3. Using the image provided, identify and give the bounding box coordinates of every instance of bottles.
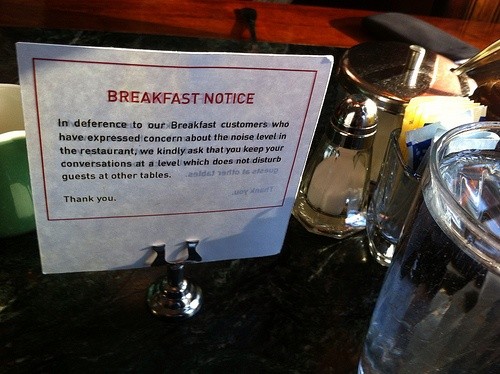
[301,93,378,218]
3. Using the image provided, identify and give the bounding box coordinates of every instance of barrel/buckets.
[357,119,500,374]
[331,39,467,198]
[366,127,438,267]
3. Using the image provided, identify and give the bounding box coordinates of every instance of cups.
[0,83,33,234]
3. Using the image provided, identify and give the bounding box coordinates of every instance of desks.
[0,0,500,51]
